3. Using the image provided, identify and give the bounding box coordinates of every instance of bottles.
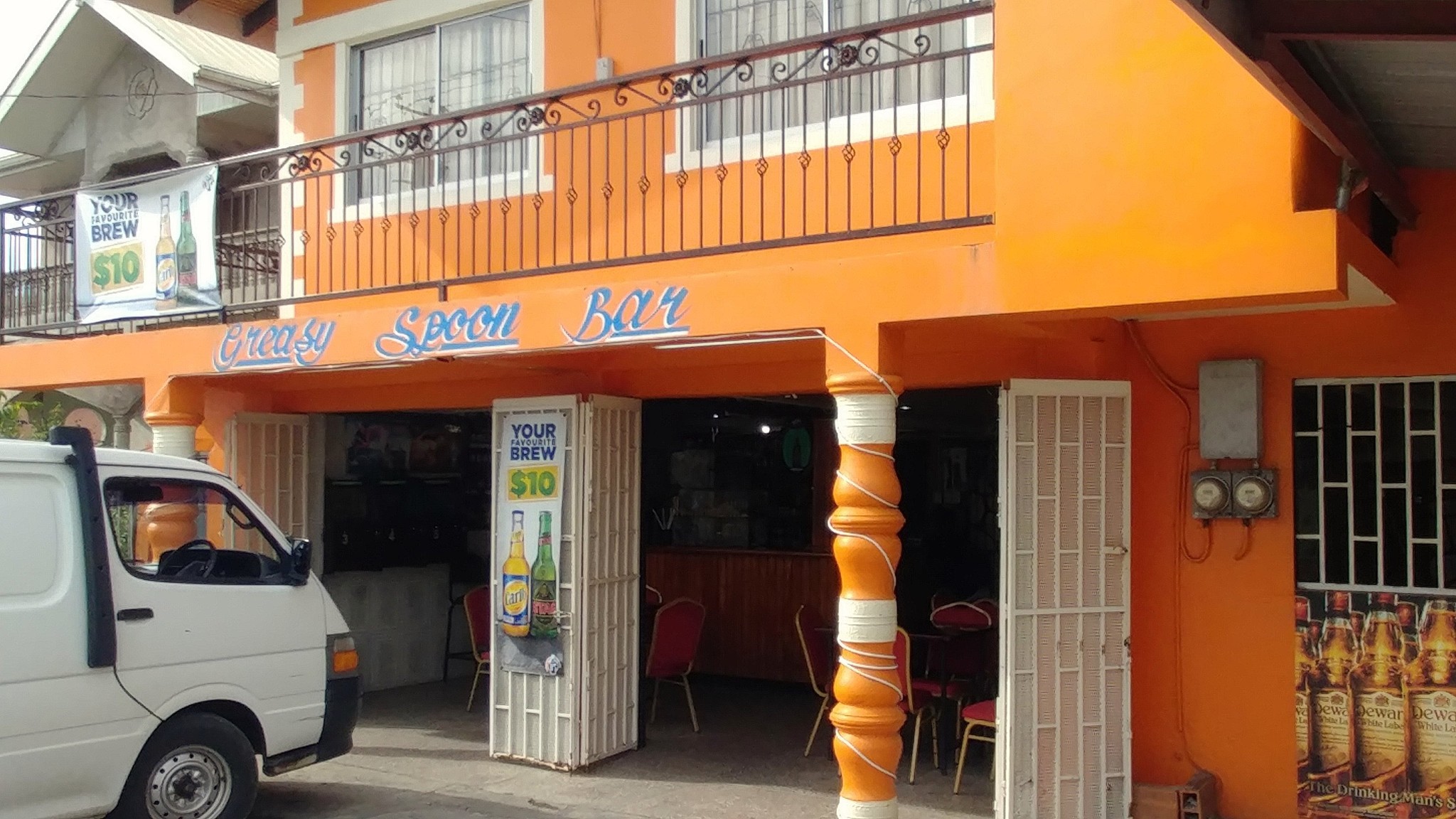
[155,195,177,310]
[1397,600,1420,663]
[530,510,557,638]
[1311,592,1352,810]
[1350,612,1365,664]
[502,510,529,637]
[1294,595,1311,794]
[1401,601,1456,819]
[1311,620,1323,667]
[1347,591,1408,819]
[176,191,198,305]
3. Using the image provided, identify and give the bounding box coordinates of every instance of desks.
[815,625,996,775]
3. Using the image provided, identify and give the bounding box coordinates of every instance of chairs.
[796,603,830,757]
[463,584,490,711]
[645,597,706,731]
[892,626,939,785]
[929,603,986,765]
[645,585,663,653]
[953,695,999,795]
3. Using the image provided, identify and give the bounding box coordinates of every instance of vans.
[0,426,362,819]
